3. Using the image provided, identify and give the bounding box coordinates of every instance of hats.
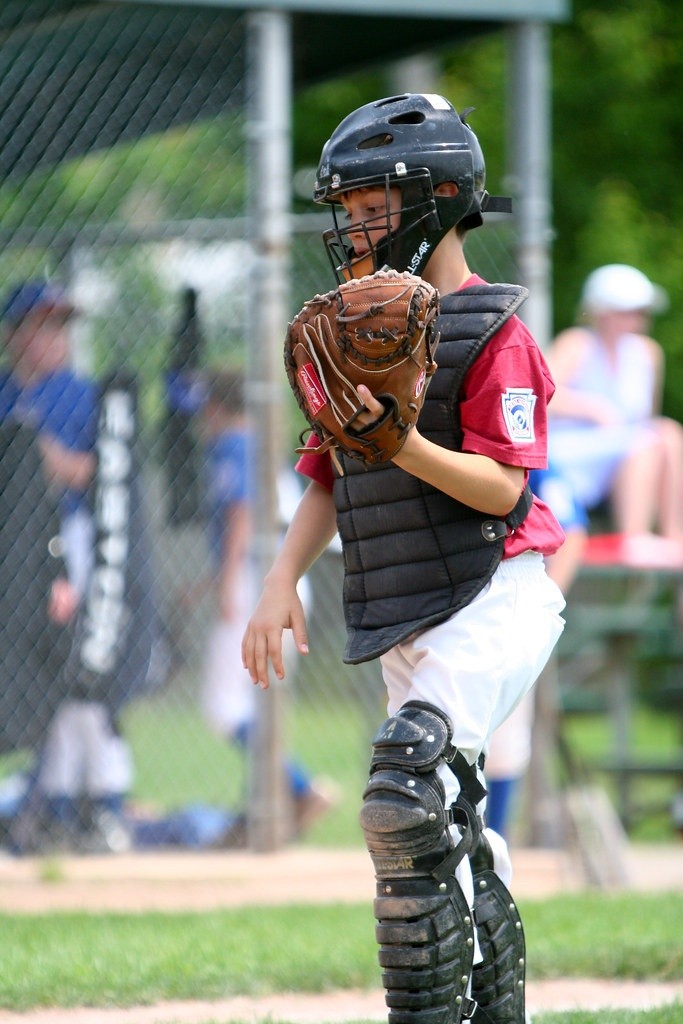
[584,264,655,311]
[0,280,82,324]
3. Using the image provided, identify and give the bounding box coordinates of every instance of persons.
[177,369,336,829]
[484,263,683,845]
[0,278,139,850]
[241,93,565,1024]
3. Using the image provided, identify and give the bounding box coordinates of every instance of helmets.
[314,92,513,287]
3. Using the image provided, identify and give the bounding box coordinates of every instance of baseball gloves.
[278,273,434,463]
